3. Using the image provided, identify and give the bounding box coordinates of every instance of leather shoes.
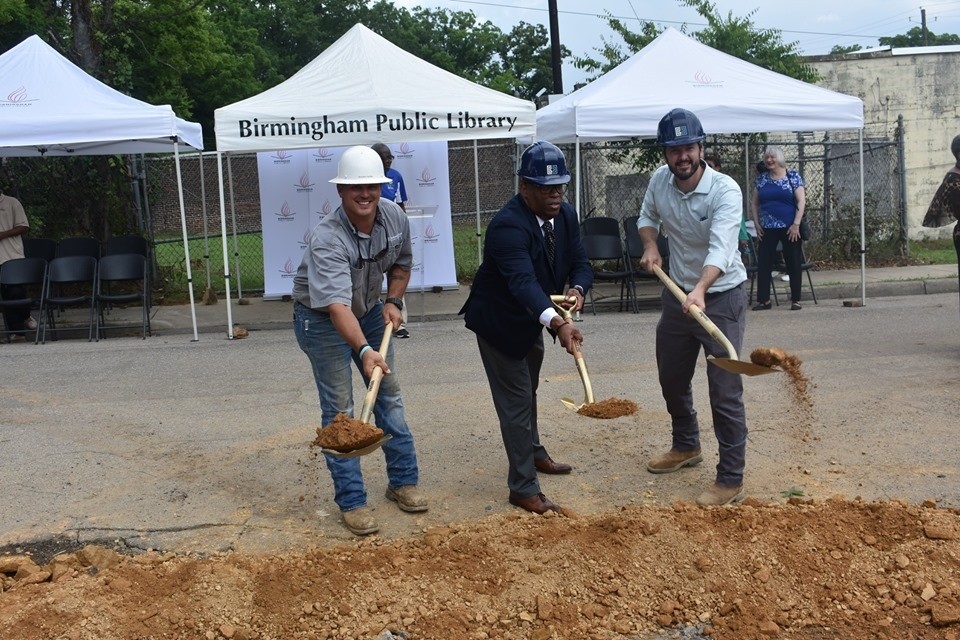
[508,491,559,515]
[536,456,571,474]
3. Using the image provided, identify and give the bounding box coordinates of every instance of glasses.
[536,183,568,194]
[383,155,395,160]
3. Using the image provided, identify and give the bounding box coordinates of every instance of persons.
[751,147,806,311]
[457,142,596,512]
[292,146,429,534]
[635,108,748,506]
[0,186,37,340]
[922,135,960,303]
[371,143,410,338]
[705,156,750,259]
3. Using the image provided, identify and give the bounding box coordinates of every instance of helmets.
[657,108,706,147]
[328,144,393,185]
[516,141,571,185]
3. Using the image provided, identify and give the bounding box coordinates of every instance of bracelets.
[359,344,372,359]
[545,322,569,344]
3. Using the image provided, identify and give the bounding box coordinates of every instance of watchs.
[385,298,403,310]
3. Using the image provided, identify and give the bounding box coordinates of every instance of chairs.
[744,220,819,308]
[568,216,637,316]
[56,235,105,315]
[41,254,102,346]
[622,216,670,314]
[94,252,152,342]
[0,256,56,345]
[23,237,59,316]
[102,234,152,316]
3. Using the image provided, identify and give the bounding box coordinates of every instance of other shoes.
[343,506,379,535]
[25,316,37,329]
[752,302,771,310]
[647,447,703,473]
[696,486,745,507]
[393,329,410,338]
[791,302,802,310]
[386,484,429,512]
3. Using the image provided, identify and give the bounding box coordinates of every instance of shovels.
[549,295,638,420]
[652,261,779,377]
[320,318,392,455]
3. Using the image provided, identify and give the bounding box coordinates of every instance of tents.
[214,22,538,341]
[515,28,866,323]
[0,34,217,342]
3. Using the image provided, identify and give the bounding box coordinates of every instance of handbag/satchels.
[800,215,811,241]
